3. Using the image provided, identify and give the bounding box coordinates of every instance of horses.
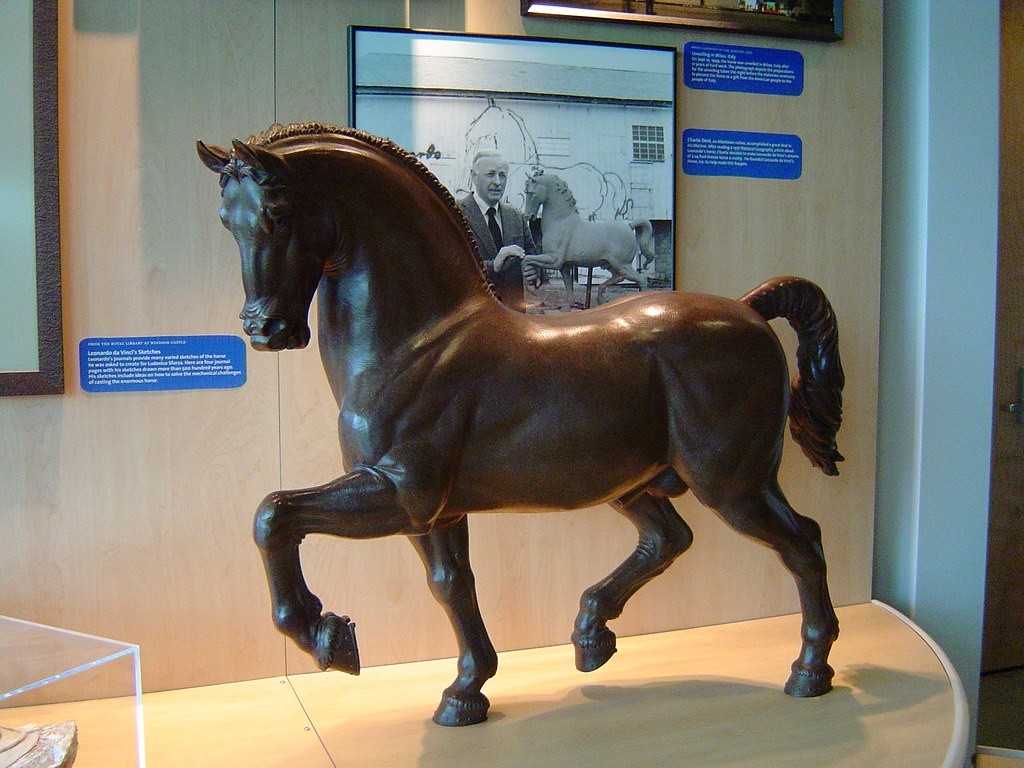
[193,121,848,728]
[523,170,654,310]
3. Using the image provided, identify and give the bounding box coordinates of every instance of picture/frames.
[0,0,72,398]
[519,0,844,46]
[346,24,678,318]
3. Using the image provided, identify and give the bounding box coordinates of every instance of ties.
[485,208,503,253]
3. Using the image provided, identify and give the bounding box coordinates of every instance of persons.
[458,151,544,314]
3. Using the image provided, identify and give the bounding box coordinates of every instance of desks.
[0,598,976,768]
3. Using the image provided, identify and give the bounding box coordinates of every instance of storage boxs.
[0,614,148,768]
